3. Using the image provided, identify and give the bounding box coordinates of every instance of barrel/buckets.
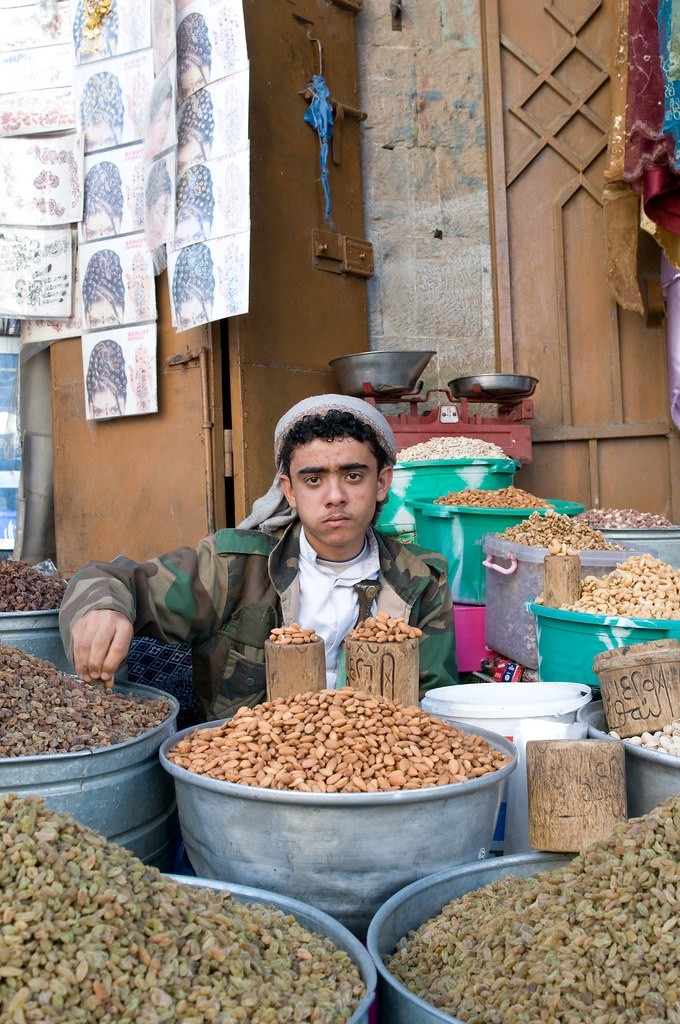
[0,459,680,1024]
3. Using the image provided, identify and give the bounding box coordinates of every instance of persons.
[59,393,460,724]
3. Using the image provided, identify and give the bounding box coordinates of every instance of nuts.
[0,433,680,1024]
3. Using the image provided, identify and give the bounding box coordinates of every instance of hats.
[273,394,397,481]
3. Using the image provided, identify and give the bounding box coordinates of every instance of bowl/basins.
[327,350,437,397]
[447,373,539,399]
